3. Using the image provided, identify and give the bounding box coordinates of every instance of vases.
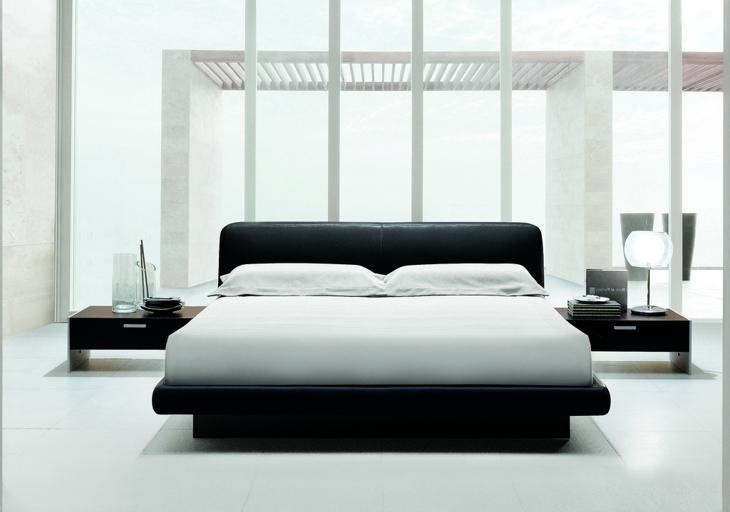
[110,252,156,315]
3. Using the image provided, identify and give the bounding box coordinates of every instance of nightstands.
[552,307,693,376]
[65,305,208,373]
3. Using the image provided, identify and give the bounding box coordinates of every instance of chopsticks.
[139,240,152,304]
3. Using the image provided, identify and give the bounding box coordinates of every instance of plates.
[574,296,611,304]
[140,304,183,315]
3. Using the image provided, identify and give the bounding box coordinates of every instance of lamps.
[623,230,673,317]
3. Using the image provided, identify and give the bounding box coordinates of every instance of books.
[566,298,623,318]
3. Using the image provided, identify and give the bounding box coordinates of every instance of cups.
[136,260,157,310]
[110,253,137,315]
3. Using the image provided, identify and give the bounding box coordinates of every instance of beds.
[151,223,610,452]
